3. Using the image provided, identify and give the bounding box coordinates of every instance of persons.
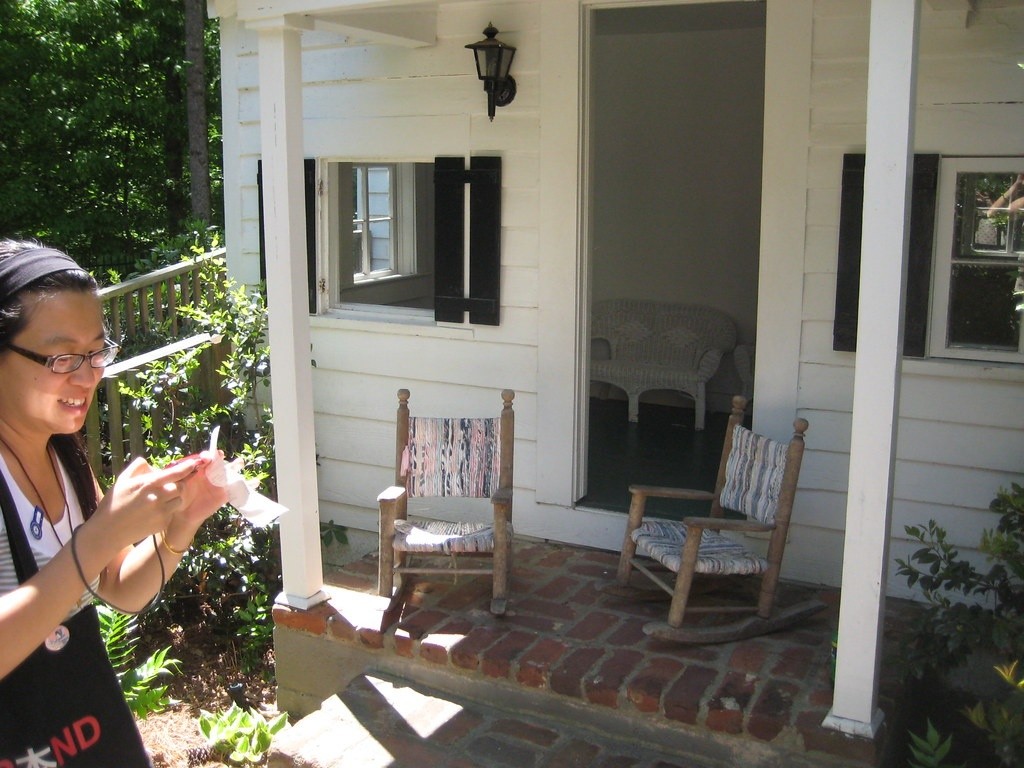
[0,243,234,768]
[986,174,1024,294]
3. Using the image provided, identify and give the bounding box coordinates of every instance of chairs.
[735,344,754,411]
[376,389,516,616]
[603,396,829,643]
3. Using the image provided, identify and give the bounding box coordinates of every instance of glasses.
[0,336,122,374]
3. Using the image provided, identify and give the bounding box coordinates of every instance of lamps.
[464,21,517,121]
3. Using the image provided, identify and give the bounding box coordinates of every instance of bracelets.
[1000,191,1010,203]
[159,529,195,556]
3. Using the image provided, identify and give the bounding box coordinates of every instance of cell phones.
[166,457,212,479]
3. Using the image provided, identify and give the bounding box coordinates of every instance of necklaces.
[0,438,73,548]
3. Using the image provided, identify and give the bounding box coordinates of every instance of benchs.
[590,298,739,433]
